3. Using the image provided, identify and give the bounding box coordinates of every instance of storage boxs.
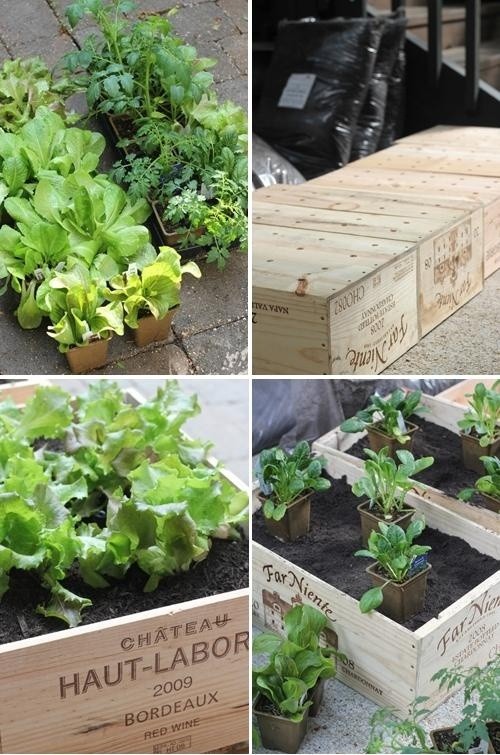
[432,379,500,417]
[252,448,500,723]
[348,143,500,278]
[396,122,500,141]
[253,184,420,375]
[304,166,484,341]
[311,389,500,539]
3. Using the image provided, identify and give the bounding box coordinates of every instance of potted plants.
[352,446,435,551]
[253,602,348,717]
[338,385,431,466]
[0,386,249,753]
[456,379,499,477]
[254,441,332,541]
[355,517,433,624]
[252,653,313,753]
[459,453,499,512]
